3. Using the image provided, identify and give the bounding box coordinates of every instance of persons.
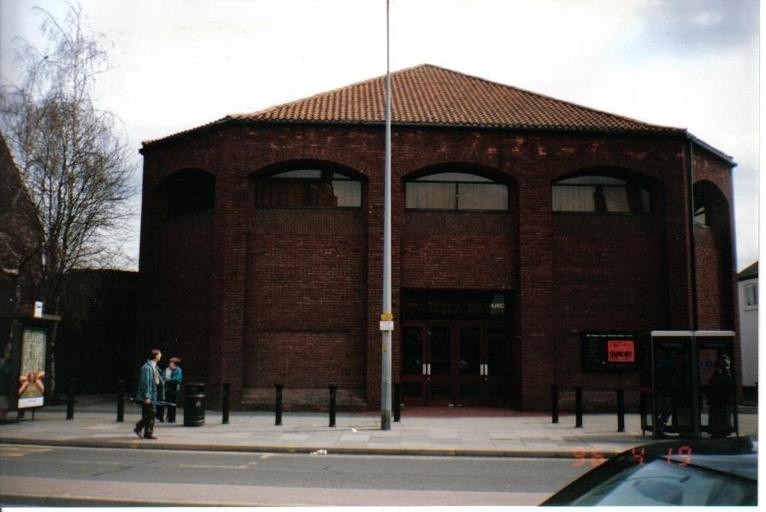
[160,357,183,422]
[133,349,162,439]
[18,371,46,396]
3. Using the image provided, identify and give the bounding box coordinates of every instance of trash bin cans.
[183,383,207,426]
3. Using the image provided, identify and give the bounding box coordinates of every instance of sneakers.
[133,428,158,439]
